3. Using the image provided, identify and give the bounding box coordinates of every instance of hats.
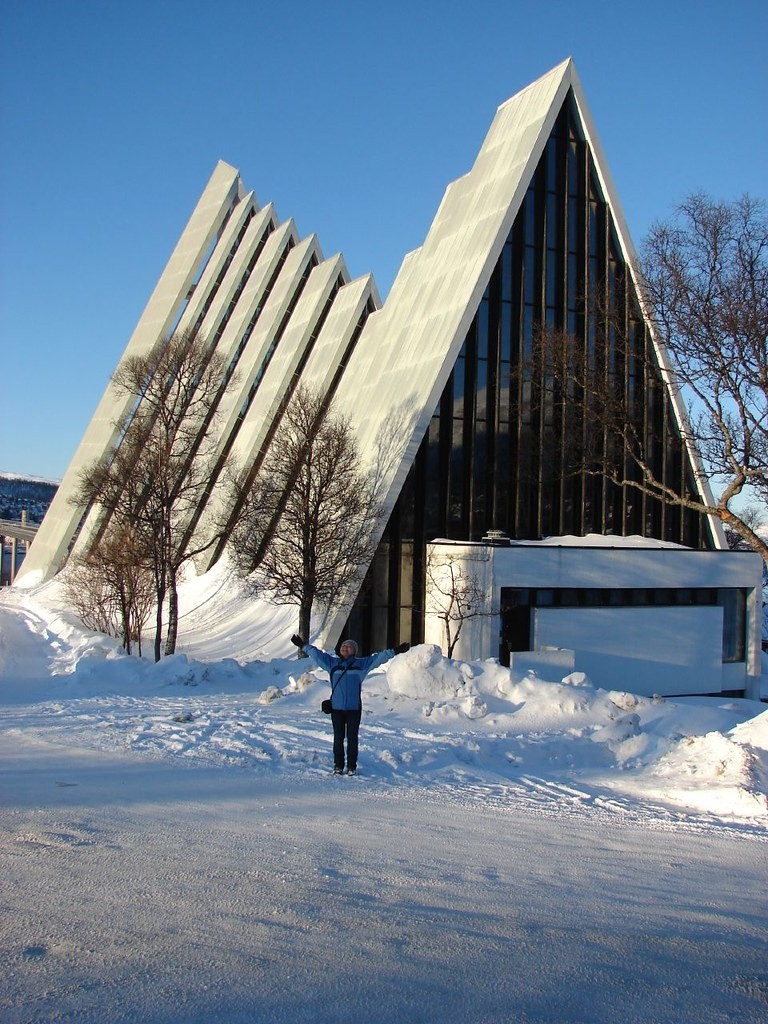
[342,639,358,657]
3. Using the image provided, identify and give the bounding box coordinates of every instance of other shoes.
[347,768,356,775]
[332,764,343,778]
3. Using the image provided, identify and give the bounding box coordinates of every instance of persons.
[291,634,410,775]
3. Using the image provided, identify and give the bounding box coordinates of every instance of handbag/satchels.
[321,699,332,714]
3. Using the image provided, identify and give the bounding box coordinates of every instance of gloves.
[290,634,303,648]
[393,641,410,655]
